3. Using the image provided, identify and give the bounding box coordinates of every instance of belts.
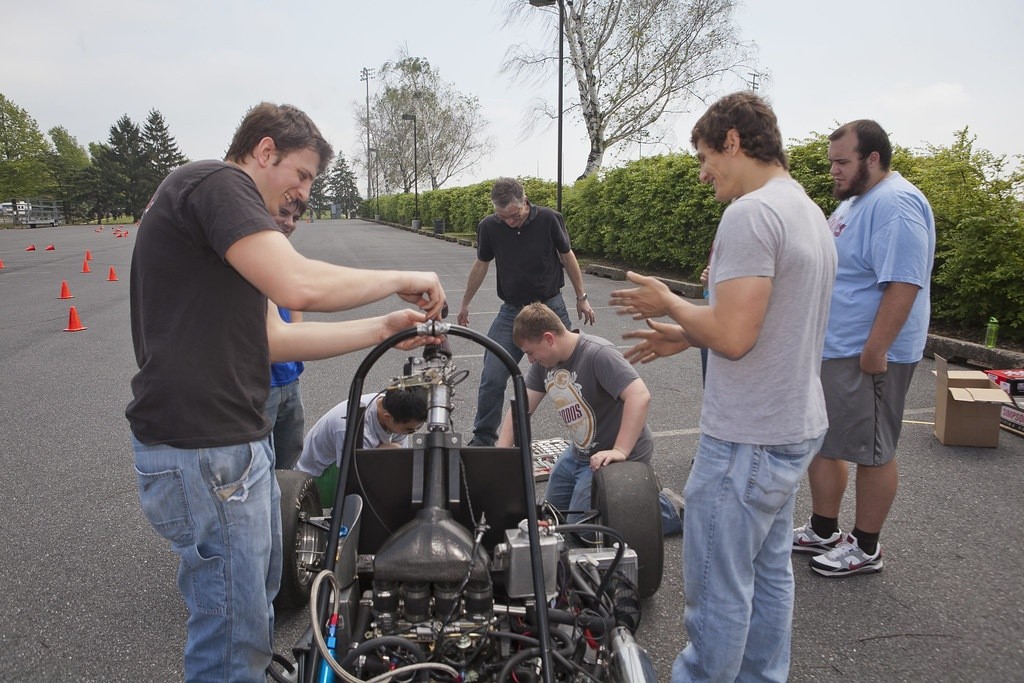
[505,291,561,309]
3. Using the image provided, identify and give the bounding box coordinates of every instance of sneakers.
[792,515,843,555]
[808,532,883,577]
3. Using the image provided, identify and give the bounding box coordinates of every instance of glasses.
[494,208,521,219]
[397,422,424,435]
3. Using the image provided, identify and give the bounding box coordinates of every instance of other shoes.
[467,435,494,446]
[662,487,685,531]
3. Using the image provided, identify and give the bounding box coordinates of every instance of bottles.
[985,316,999,347]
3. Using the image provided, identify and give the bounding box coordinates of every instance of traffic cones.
[120,224,123,227]
[85,249,94,260]
[62,306,88,332]
[124,230,128,234]
[80,259,92,273]
[57,280,74,299]
[113,229,121,234]
[124,232,126,236]
[46,244,55,250]
[107,266,119,281]
[0,259,4,269]
[117,233,122,237]
[95,224,103,232]
[25,245,35,251]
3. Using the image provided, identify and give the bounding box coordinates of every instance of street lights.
[370,148,379,215]
[361,67,376,199]
[529,0,564,214]
[402,114,418,220]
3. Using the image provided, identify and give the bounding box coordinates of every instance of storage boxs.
[983,368,1024,395]
[930,352,1013,447]
[1000,396,1024,435]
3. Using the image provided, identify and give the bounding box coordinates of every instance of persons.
[266,198,308,469]
[496,299,686,545]
[790,119,936,579]
[700,266,710,390]
[457,178,595,446]
[293,385,429,510]
[609,92,836,683]
[125,101,334,683]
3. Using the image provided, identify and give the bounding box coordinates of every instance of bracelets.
[576,293,587,301]
